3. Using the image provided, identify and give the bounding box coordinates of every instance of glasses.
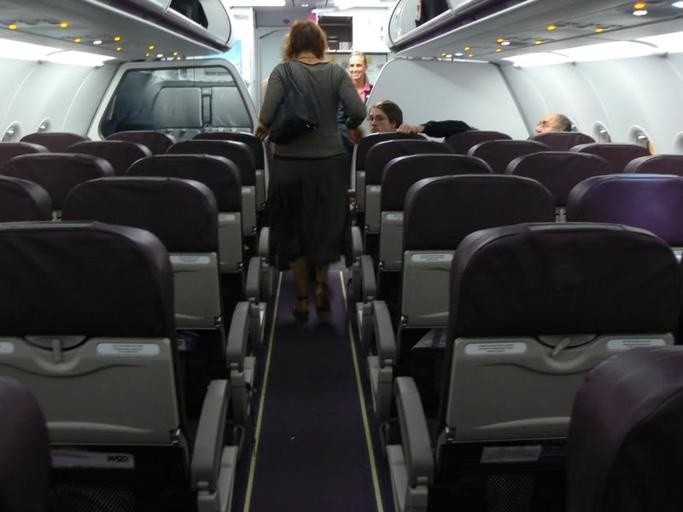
[367,117,387,121]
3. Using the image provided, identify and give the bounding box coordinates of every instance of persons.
[534,113,571,133]
[396,119,479,138]
[307,100,354,280]
[254,19,366,316]
[346,50,372,106]
[366,99,403,132]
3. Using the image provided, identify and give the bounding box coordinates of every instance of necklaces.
[295,54,320,63]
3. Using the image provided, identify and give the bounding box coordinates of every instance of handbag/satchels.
[268,85,319,145]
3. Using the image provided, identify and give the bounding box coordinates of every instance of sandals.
[293,295,310,322]
[315,281,331,309]
